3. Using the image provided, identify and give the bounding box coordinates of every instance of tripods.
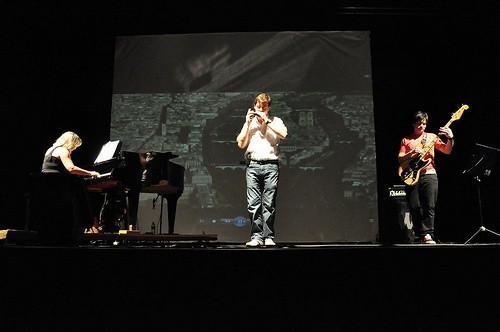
[465,150,500,245]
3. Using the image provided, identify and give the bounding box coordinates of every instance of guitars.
[397,103,469,186]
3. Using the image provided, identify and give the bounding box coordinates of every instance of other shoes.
[420,234,436,243]
[245,233,264,246]
[265,238,276,245]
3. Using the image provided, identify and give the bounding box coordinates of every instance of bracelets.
[448,137,454,140]
[265,120,271,125]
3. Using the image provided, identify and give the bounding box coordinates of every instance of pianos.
[76,140,184,235]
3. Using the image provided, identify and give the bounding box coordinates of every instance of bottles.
[151,222,155,234]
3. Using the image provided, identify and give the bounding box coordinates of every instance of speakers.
[384,196,417,244]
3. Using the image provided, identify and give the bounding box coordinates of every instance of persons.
[43,131,109,233]
[237,93,287,245]
[399,111,454,243]
